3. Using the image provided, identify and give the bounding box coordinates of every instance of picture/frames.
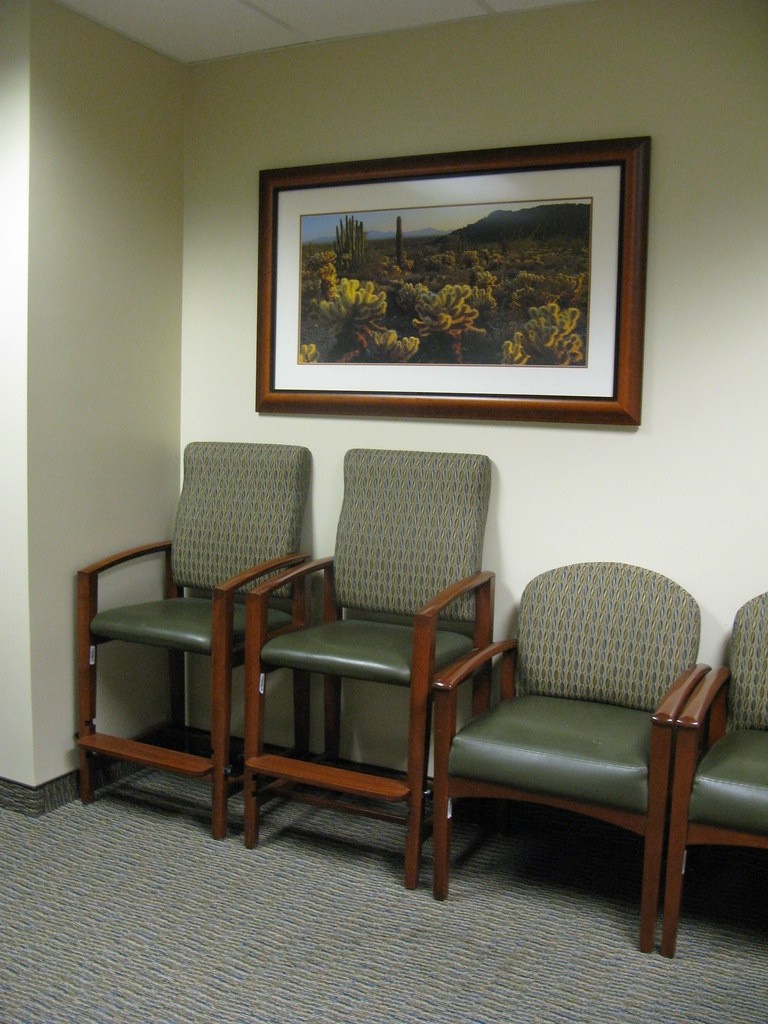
[253,137,650,427]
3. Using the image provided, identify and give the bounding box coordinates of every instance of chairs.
[429,561,713,953]
[243,449,494,894]
[73,440,313,840]
[663,586,768,958]
